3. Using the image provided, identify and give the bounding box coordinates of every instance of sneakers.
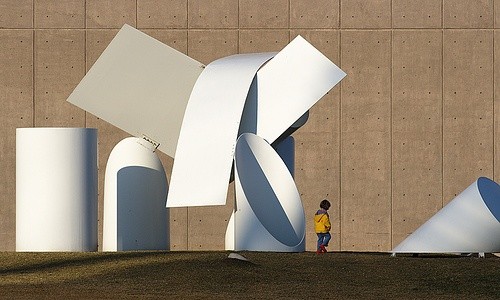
[320,245,327,252]
[317,251,325,254]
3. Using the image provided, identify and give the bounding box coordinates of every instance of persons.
[313,199,332,254]
